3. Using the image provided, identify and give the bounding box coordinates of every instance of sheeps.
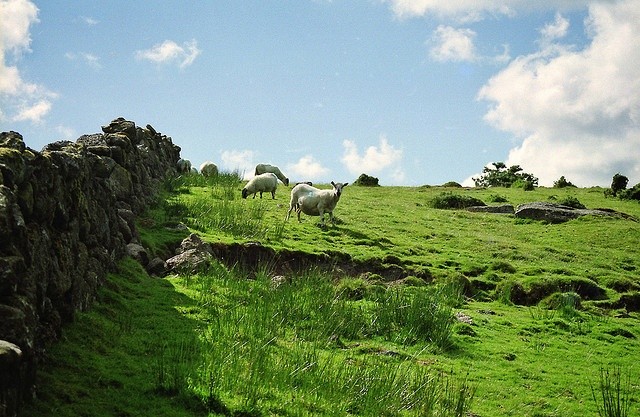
[242,173,278,199]
[255,164,289,186]
[176,159,191,175]
[286,181,348,229]
[200,161,218,177]
